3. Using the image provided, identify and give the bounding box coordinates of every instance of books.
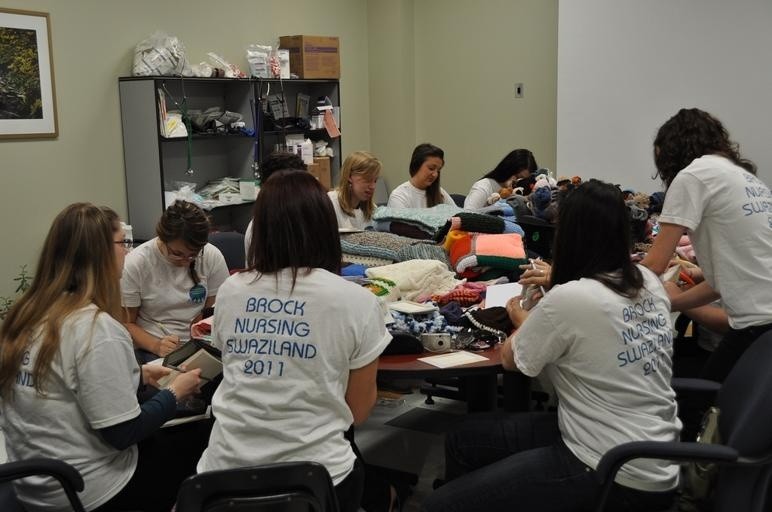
[154,346,222,404]
[163,175,260,210]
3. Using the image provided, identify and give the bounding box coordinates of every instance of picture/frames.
[0,8,59,141]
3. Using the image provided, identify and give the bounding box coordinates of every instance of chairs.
[171,462,339,512]
[0,457,86,512]
[581,331,772,512]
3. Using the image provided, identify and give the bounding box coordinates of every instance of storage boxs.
[281,35,340,79]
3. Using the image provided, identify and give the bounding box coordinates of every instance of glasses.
[163,240,206,266]
[113,237,134,250]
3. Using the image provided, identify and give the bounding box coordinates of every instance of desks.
[197,283,695,464]
[308,156,332,194]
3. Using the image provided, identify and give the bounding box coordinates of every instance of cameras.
[422,332,451,351]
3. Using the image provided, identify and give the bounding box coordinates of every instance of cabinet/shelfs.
[121,76,343,251]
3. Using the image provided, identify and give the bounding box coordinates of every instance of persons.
[415,179,680,507]
[635,108,772,422]
[243,152,314,268]
[462,149,540,213]
[326,151,387,234]
[384,143,459,214]
[119,202,229,356]
[665,264,736,378]
[192,169,393,508]
[0,204,204,507]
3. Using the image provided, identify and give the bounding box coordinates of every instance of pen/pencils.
[680,272,696,288]
[528,258,545,296]
[157,322,170,335]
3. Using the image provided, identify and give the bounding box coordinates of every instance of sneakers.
[378,373,411,395]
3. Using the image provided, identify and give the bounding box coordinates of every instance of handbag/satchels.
[681,405,723,503]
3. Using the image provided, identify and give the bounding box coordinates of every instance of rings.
[538,269,545,277]
